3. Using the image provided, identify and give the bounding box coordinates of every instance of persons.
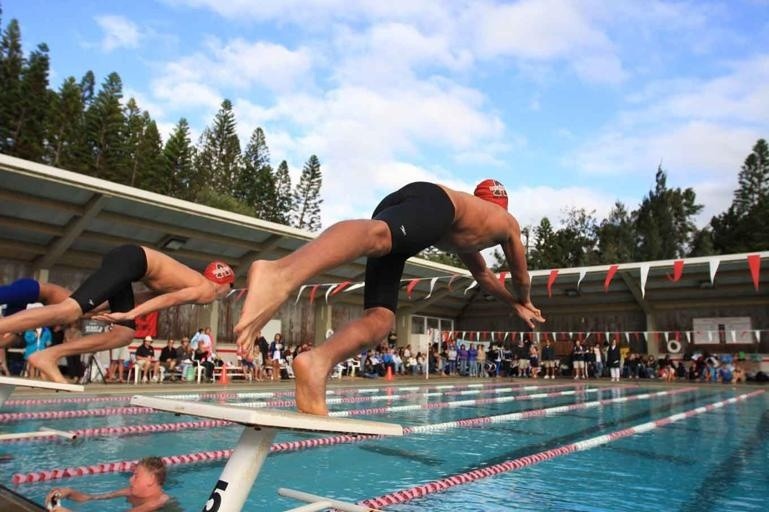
[0,301,85,382]
[44,456,181,511]
[0,277,112,349]
[232,178,547,417]
[102,328,750,386]
[0,243,236,393]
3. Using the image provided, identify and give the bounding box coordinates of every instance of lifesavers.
[667,340,681,353]
[326,329,334,338]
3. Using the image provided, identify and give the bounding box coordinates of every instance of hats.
[204,261,236,284]
[475,179,508,211]
[143,336,153,343]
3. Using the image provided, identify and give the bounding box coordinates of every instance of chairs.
[127,352,361,385]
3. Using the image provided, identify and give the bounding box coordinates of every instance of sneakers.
[142,375,180,384]
[512,372,556,379]
[105,376,127,383]
[252,375,282,383]
[610,378,618,382]
[205,376,214,384]
[441,371,489,378]
[573,374,586,380]
